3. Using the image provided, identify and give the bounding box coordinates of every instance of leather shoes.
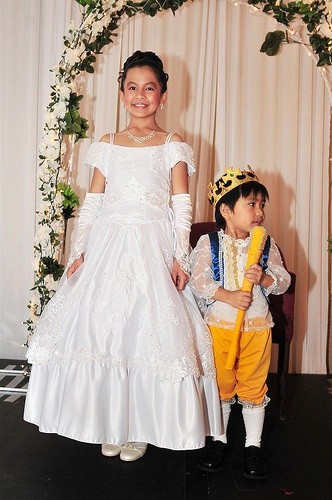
[199,440,229,473]
[242,446,269,479]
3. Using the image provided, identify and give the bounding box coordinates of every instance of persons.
[189,165,291,480]
[22,50,224,462]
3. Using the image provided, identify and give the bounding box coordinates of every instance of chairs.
[190,222,296,421]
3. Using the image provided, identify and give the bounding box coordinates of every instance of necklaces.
[127,126,158,144]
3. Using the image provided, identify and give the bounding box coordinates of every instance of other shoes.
[102,443,122,456]
[120,442,148,462]
